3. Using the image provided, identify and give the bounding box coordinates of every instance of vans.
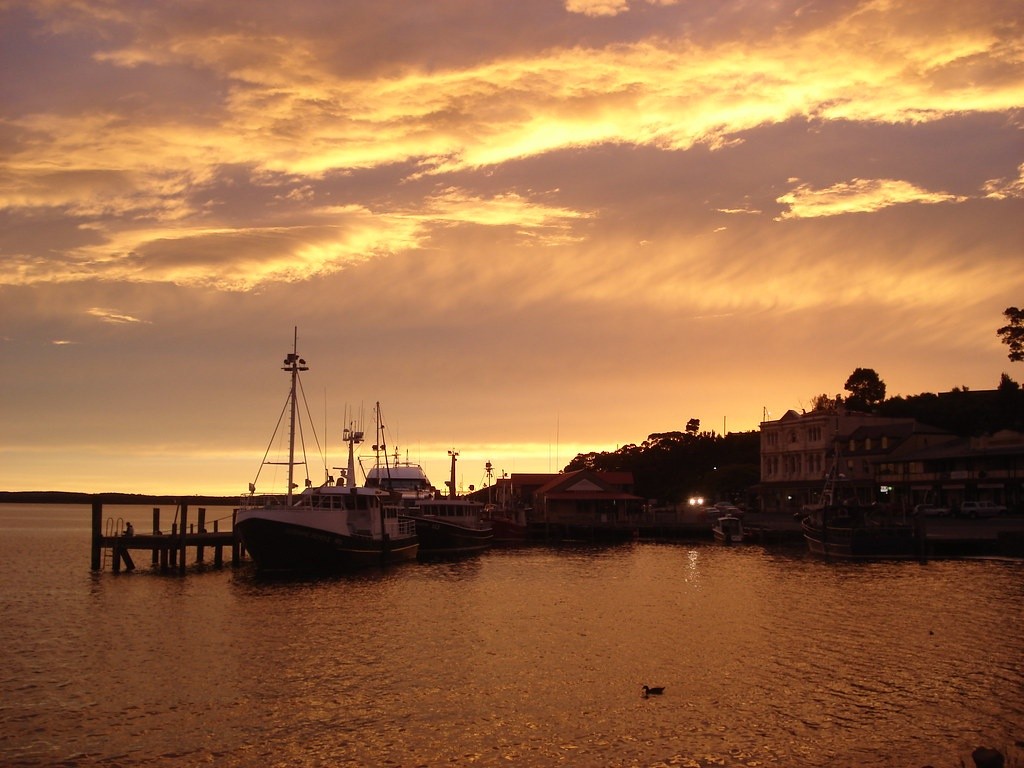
[960,501,1008,520]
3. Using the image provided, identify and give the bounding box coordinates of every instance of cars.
[912,504,949,519]
[697,501,744,523]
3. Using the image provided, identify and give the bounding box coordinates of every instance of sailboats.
[358,402,494,541]
[232,325,419,569]
[465,460,533,538]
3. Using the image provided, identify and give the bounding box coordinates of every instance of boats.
[712,514,745,544]
[800,418,928,558]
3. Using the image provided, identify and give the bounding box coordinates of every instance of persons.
[122,522,134,538]
